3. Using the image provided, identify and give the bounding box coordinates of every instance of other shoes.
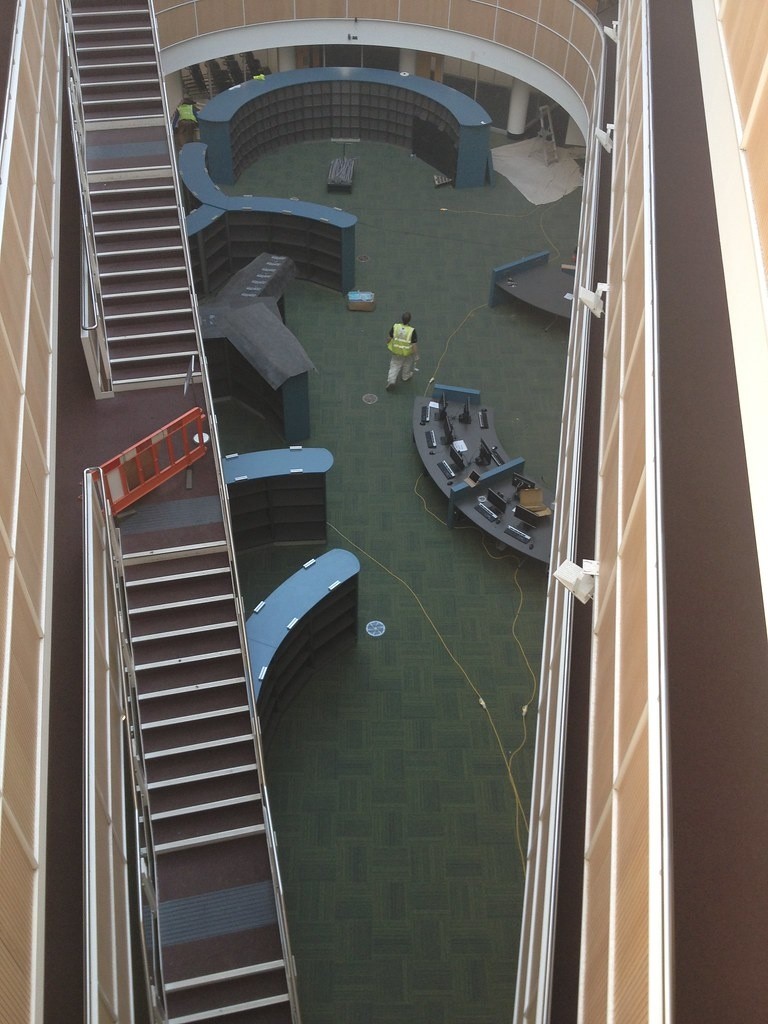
[402,372,413,380]
[386,381,395,390]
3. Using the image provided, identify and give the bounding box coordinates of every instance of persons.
[172,99,201,151]
[386,310,420,393]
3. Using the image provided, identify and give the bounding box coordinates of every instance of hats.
[403,312,411,324]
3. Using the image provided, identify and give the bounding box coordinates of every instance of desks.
[412,385,556,564]
[492,250,575,332]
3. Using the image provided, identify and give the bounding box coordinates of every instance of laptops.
[464,470,481,488]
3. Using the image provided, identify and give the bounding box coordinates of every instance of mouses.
[529,544,533,549]
[497,520,500,524]
[447,481,453,485]
[420,422,426,425]
[429,451,435,455]
[491,445,497,450]
[481,408,487,412]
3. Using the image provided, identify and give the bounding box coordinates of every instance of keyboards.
[426,430,436,448]
[421,406,431,422]
[437,460,456,480]
[504,524,531,544]
[479,412,489,429]
[474,503,498,523]
[491,450,505,467]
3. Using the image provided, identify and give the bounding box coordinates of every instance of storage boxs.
[347,292,375,311]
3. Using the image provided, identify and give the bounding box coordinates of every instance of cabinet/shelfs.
[245,547,361,758]
[222,445,334,551]
[178,67,491,443]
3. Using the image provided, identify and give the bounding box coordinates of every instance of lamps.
[579,283,609,318]
[594,123,615,153]
[603,20,618,43]
[553,559,599,604]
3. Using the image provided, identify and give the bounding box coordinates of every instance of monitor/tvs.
[434,393,540,531]
